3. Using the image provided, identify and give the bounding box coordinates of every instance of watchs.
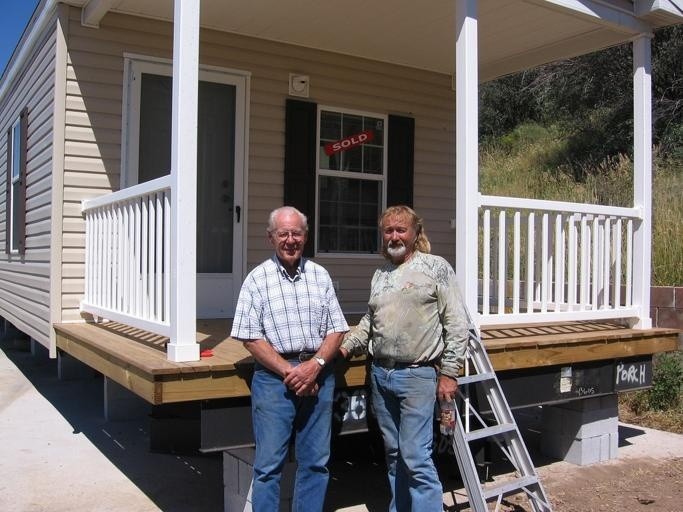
[313,356,327,367]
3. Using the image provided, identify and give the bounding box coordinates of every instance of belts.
[279,351,316,363]
[377,358,432,369]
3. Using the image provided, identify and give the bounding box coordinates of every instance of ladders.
[453,303,552,512]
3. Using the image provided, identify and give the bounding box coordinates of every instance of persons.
[229,204,351,512]
[338,204,472,512]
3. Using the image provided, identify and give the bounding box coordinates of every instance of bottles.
[440,397,456,436]
